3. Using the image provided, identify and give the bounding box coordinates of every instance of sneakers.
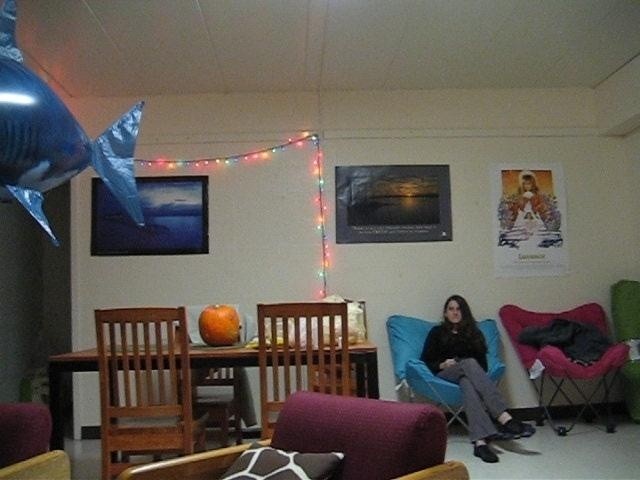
[503,418,536,438]
[473,443,499,463]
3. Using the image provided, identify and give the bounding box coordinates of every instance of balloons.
[0,0,146,248]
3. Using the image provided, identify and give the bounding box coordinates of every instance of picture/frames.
[91,176,209,258]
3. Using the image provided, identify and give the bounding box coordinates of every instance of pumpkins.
[198,301,242,347]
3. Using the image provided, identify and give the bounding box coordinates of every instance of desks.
[48,342,379,453]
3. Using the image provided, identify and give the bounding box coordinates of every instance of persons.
[508,173,549,232]
[419,294,536,465]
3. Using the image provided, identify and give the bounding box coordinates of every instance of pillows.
[219,433,349,480]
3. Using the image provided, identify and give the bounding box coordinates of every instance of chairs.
[499,304,628,436]
[112,389,472,480]
[258,304,349,441]
[387,313,510,435]
[311,298,368,397]
[1,399,73,479]
[611,273,639,426]
[93,308,208,480]
[173,304,244,447]
